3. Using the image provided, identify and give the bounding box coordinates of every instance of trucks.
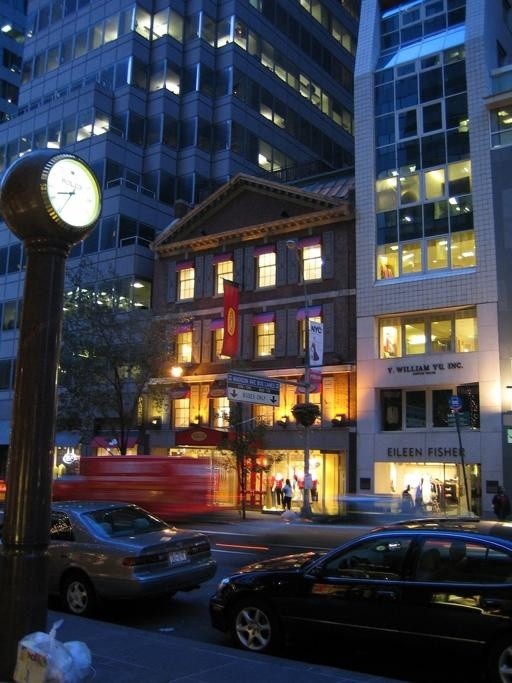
[54,455,221,525]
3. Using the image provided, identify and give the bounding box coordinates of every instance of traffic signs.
[227,372,281,408]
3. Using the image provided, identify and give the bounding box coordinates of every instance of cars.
[336,495,478,521]
[208,520,512,682]
[0,500,218,617]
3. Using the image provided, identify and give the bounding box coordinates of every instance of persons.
[492,486,510,521]
[310,476,320,505]
[402,485,414,513]
[272,475,284,505]
[415,478,428,516]
[281,479,294,509]
[295,473,304,502]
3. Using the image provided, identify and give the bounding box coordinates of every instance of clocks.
[39,153,102,234]
